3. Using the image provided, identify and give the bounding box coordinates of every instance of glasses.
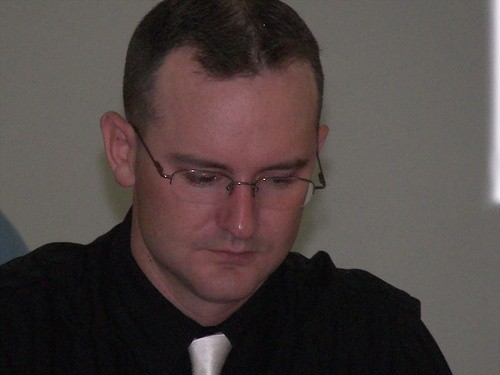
[129,121,326,208]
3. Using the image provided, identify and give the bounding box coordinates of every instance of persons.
[0,0,454,375]
